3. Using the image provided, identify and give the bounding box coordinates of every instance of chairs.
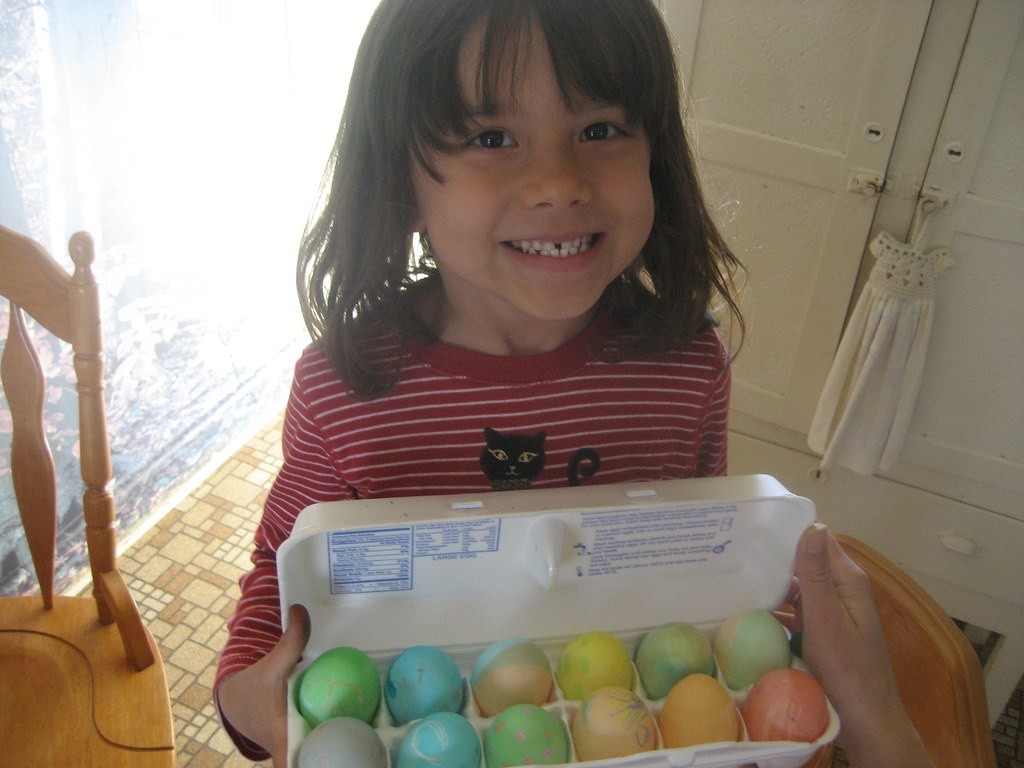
[776,533,997,768]
[0,225,175,768]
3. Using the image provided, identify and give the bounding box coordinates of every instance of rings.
[793,590,802,606]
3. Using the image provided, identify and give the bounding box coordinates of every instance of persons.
[212,0,753,768]
[772,523,935,768]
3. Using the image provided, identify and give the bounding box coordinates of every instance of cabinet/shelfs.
[661,0,1024,732]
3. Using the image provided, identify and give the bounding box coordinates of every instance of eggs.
[297,608,829,767]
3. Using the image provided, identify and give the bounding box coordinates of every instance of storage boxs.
[272,472,840,768]
